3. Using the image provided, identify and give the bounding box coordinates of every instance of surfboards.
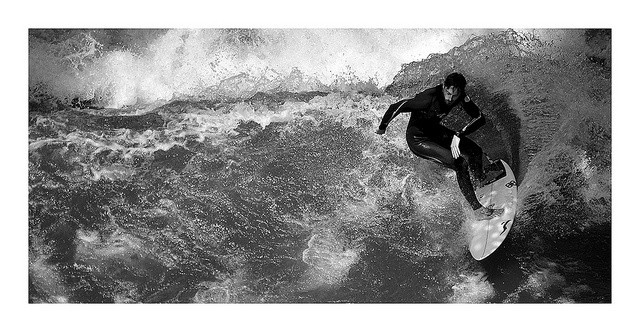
[470,160,518,261]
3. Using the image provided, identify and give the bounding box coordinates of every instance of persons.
[374,72,504,220]
